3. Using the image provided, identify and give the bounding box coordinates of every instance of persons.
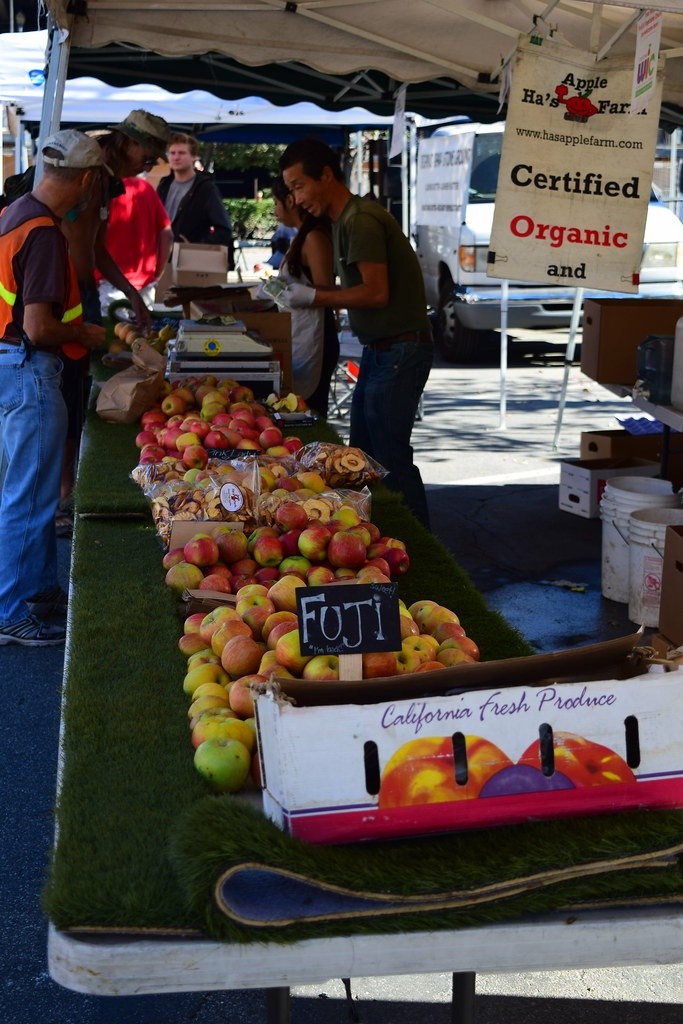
[157,131,236,271]
[1,109,155,535]
[1,129,106,648]
[272,139,432,528]
[93,174,173,317]
[250,163,340,422]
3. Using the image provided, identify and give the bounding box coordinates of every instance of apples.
[135,373,332,494]
[162,503,478,792]
[113,322,174,351]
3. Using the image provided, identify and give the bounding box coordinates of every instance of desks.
[45,501,682,1024]
[71,310,406,513]
[602,385,683,480]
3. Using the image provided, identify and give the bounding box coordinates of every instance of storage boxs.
[188,297,293,398]
[581,298,683,385]
[172,243,228,288]
[580,429,664,459]
[658,525,683,648]
[250,621,683,847]
[559,458,662,519]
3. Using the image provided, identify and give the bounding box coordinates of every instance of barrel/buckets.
[598,476,683,603]
[626,508,683,629]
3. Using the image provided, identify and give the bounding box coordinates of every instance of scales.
[179,317,275,359]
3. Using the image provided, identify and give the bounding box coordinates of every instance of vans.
[415,119,683,352]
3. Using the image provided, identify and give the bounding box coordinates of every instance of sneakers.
[1,614,66,646]
[25,584,68,611]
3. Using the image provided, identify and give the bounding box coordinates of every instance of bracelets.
[122,286,132,293]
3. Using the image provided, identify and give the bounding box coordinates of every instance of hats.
[107,109,171,164]
[41,129,115,176]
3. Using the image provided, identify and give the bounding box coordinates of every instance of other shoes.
[55,509,70,535]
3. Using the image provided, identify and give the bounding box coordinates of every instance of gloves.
[275,282,316,308]
